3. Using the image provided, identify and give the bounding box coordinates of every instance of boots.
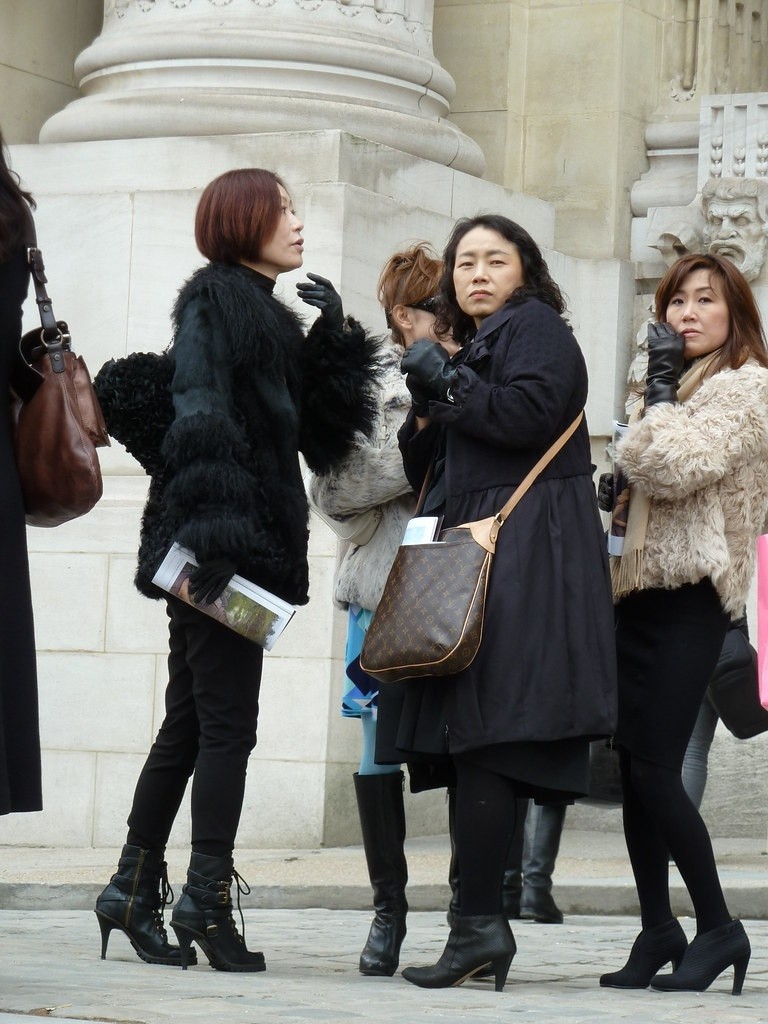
[170,852,267,972]
[501,794,525,919]
[94,845,198,967]
[401,783,517,992]
[519,797,564,924]
[353,770,409,977]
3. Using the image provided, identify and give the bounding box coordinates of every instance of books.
[152,542,297,651]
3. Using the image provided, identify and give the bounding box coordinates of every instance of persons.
[395,215,619,992]
[668,698,720,866]
[309,240,462,977]
[698,176,768,283]
[502,792,575,923]
[93,169,385,973]
[0,130,44,816]
[599,254,767,994]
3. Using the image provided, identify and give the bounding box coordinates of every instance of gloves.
[645,322,686,406]
[401,337,459,398]
[297,271,345,333]
[404,373,429,417]
[597,473,614,511]
[188,557,237,604]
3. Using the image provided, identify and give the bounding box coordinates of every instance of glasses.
[405,296,446,315]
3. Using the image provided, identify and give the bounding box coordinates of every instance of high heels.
[599,917,688,989]
[650,919,752,995]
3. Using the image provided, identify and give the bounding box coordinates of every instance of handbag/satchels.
[10,193,112,530]
[359,513,504,684]
[704,629,767,742]
